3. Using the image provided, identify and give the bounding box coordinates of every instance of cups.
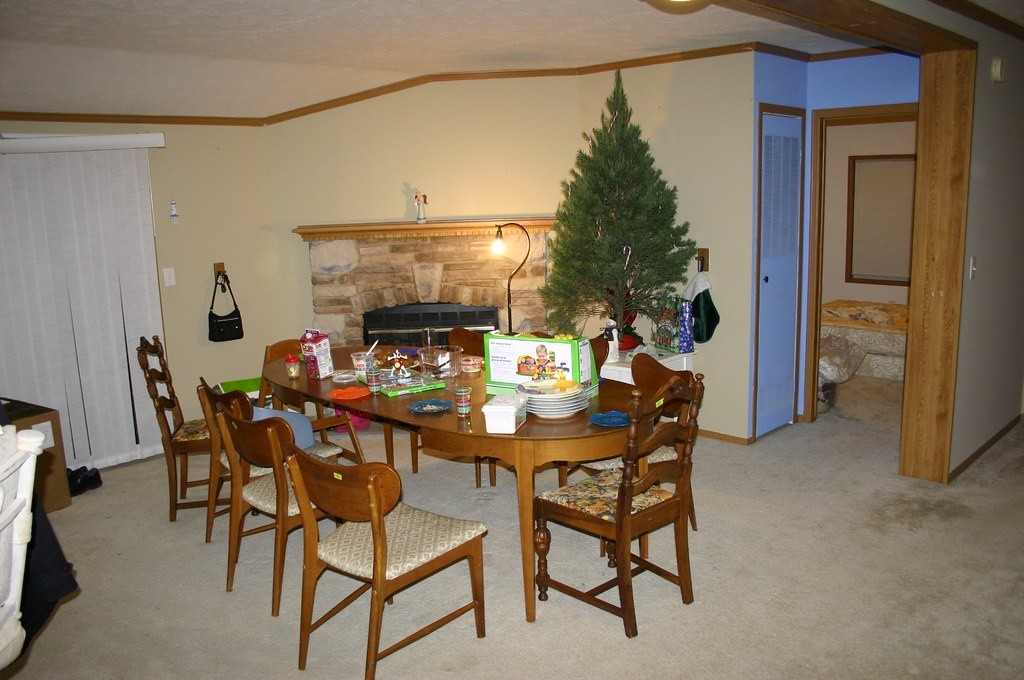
[454,383,472,418]
[350,352,374,381]
[422,326,437,346]
[285,354,300,379]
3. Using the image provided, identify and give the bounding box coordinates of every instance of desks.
[261,345,665,623]
[598,343,699,424]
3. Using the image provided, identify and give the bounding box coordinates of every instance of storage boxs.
[0,396,73,515]
[483,330,600,400]
[481,394,529,435]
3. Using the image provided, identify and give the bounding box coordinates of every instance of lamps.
[492,222,531,336]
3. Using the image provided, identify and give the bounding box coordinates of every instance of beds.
[821,299,908,384]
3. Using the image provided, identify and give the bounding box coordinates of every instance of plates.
[590,411,631,427]
[516,379,589,419]
[380,360,419,371]
[328,387,371,400]
[408,398,451,413]
[332,375,357,382]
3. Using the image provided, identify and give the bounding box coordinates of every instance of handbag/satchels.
[208,271,244,342]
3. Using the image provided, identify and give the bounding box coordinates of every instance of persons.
[415,191,426,224]
[535,344,550,365]
[605,319,619,362]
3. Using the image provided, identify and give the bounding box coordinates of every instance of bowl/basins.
[462,357,482,373]
[417,345,463,379]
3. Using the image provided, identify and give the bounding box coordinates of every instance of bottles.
[366,358,381,395]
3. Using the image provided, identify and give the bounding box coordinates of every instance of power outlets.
[968,256,976,279]
[889,301,896,304]
[162,267,177,288]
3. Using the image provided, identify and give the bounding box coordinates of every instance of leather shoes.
[67,466,103,497]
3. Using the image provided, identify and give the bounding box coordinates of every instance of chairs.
[280,439,489,680]
[136,335,251,522]
[382,325,707,639]
[197,338,351,618]
